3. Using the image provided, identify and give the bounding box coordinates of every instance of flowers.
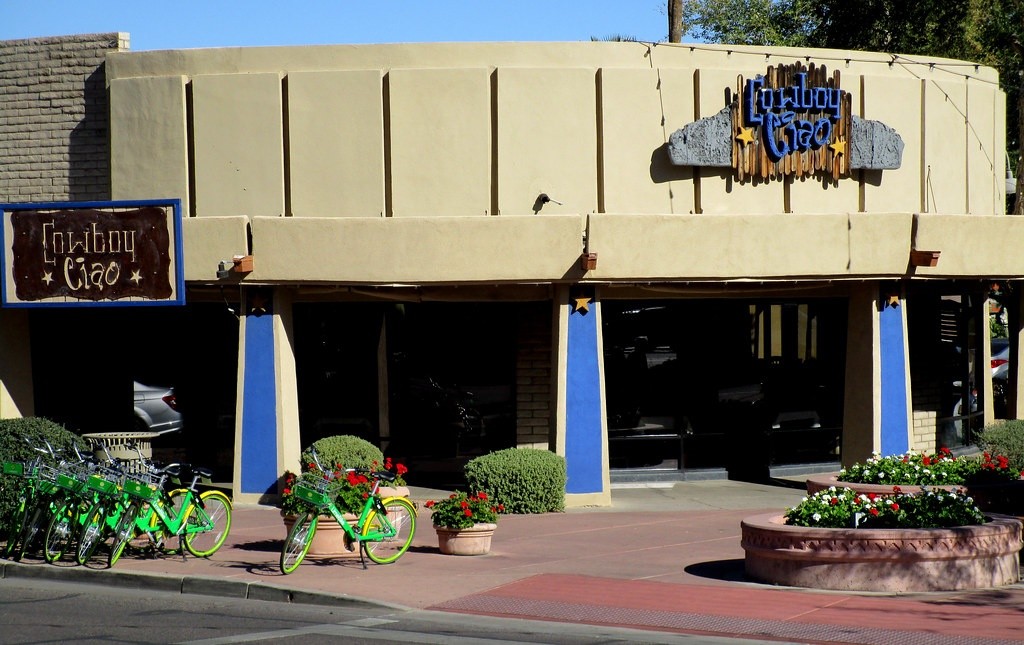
[280,462,380,519]
[369,457,408,490]
[422,489,506,530]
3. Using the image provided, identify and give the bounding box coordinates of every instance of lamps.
[216,256,254,278]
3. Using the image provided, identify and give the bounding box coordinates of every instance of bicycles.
[279,444,420,574]
[3,428,236,569]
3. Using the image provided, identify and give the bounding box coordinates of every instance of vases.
[379,486,410,541]
[432,524,497,555]
[280,510,369,559]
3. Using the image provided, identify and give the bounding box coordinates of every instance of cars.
[133,381,182,435]
[990,338,1009,380]
